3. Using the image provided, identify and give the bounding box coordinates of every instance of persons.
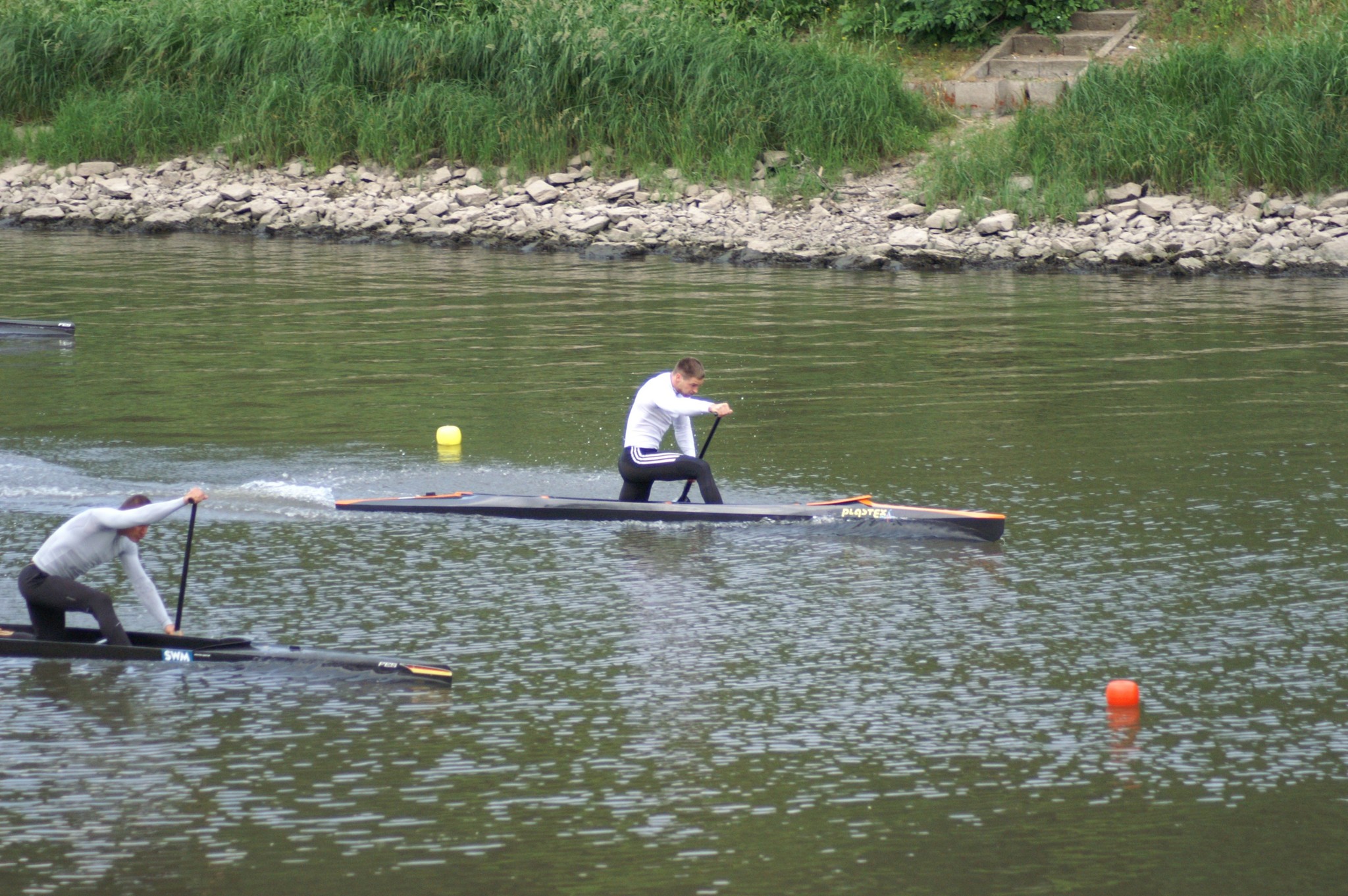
[617,357,733,504]
[18,487,208,646]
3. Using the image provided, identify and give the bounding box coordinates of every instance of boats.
[0,317,75,338]
[0,620,454,687]
[335,493,1006,542]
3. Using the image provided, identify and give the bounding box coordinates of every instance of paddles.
[677,413,722,503]
[174,497,200,631]
